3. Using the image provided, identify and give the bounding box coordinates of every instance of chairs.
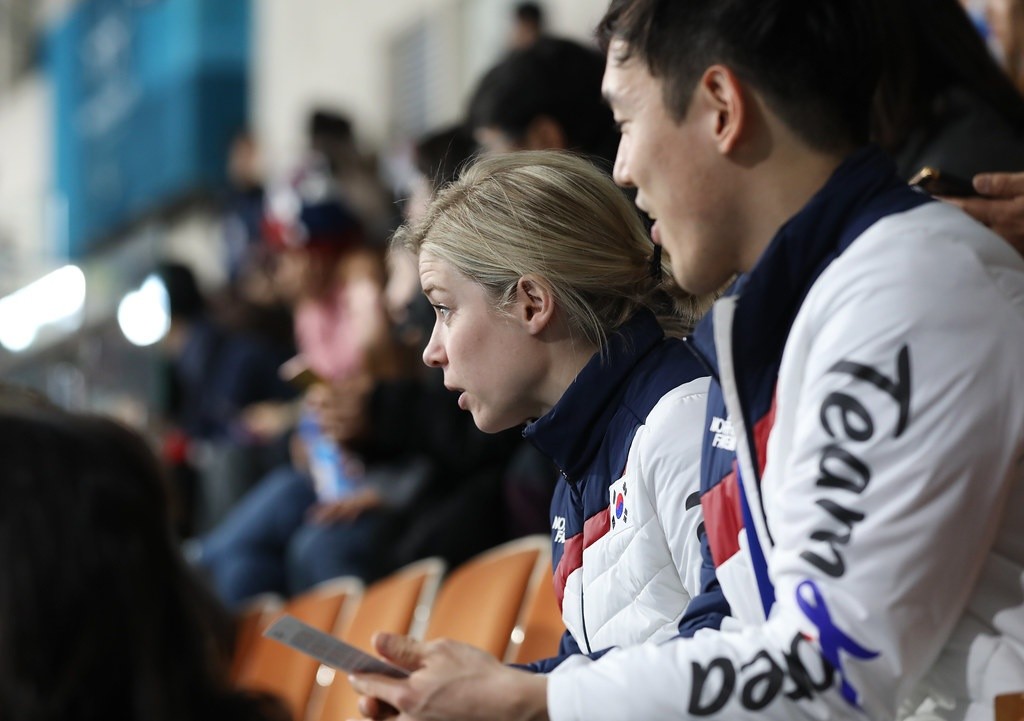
[507,552,569,669]
[420,533,551,661]
[230,591,280,685]
[237,573,363,721]
[305,556,444,721]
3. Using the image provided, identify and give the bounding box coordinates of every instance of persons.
[402,149,731,673]
[345,0,1024,721]
[0,0,1024,721]
